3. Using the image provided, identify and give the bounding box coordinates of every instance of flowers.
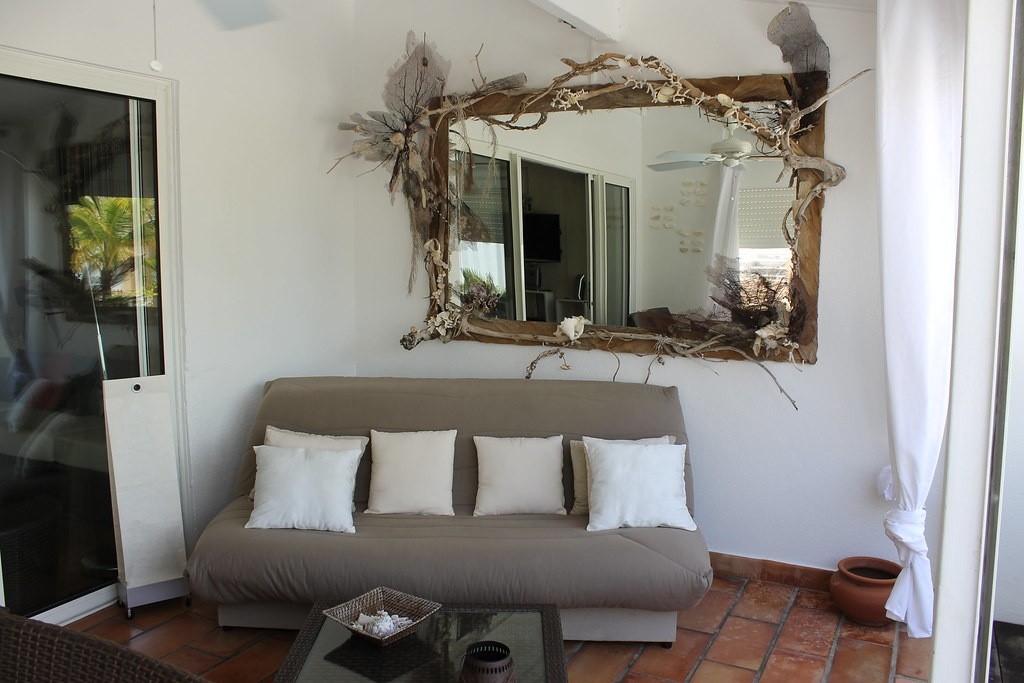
[325,1,874,411]
[18,101,164,350]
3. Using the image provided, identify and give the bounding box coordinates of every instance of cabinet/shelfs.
[525,290,556,323]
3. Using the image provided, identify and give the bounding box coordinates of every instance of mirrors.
[427,72,828,364]
[55,138,159,324]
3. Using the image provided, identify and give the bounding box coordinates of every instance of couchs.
[182,376,712,649]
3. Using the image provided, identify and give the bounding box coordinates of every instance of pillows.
[473,435,567,516]
[247,425,370,513]
[570,434,676,516]
[582,436,696,532]
[363,429,457,517]
[244,445,361,534]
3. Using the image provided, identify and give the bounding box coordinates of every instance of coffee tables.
[274,598,569,683]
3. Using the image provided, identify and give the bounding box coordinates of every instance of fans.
[643,113,786,173]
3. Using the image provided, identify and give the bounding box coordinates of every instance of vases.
[828,556,903,627]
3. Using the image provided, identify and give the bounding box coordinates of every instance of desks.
[556,298,590,324]
